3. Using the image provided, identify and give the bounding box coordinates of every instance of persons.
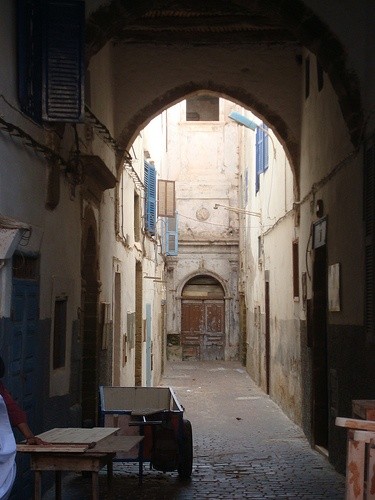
[0,356,50,500]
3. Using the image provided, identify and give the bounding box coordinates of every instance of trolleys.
[96,385,194,488]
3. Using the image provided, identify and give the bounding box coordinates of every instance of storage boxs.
[96,386,185,463]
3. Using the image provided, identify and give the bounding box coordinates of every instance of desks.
[16,427,121,500]
